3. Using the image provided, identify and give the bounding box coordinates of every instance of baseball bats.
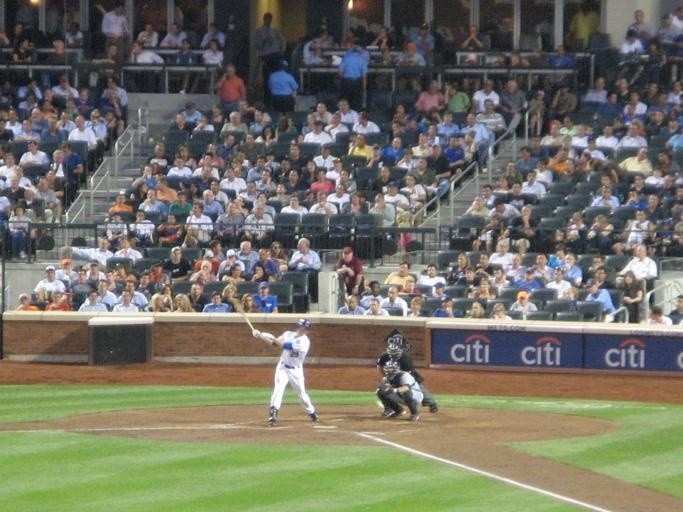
[238,305,255,332]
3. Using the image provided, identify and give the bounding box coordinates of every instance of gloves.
[252,330,261,339]
[379,382,393,394]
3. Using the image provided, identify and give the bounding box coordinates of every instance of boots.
[309,412,319,422]
[269,406,278,425]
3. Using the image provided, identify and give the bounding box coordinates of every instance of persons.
[0,0,683,325]
[383,359,424,421]
[251,319,320,425]
[376,343,438,417]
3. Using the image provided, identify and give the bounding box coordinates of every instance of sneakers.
[382,405,438,420]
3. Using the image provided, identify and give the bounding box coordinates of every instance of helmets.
[297,319,311,330]
[383,361,399,379]
[387,343,403,361]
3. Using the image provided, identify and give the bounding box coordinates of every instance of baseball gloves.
[376,384,391,396]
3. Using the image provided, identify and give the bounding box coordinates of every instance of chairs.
[1,30,683,322]
